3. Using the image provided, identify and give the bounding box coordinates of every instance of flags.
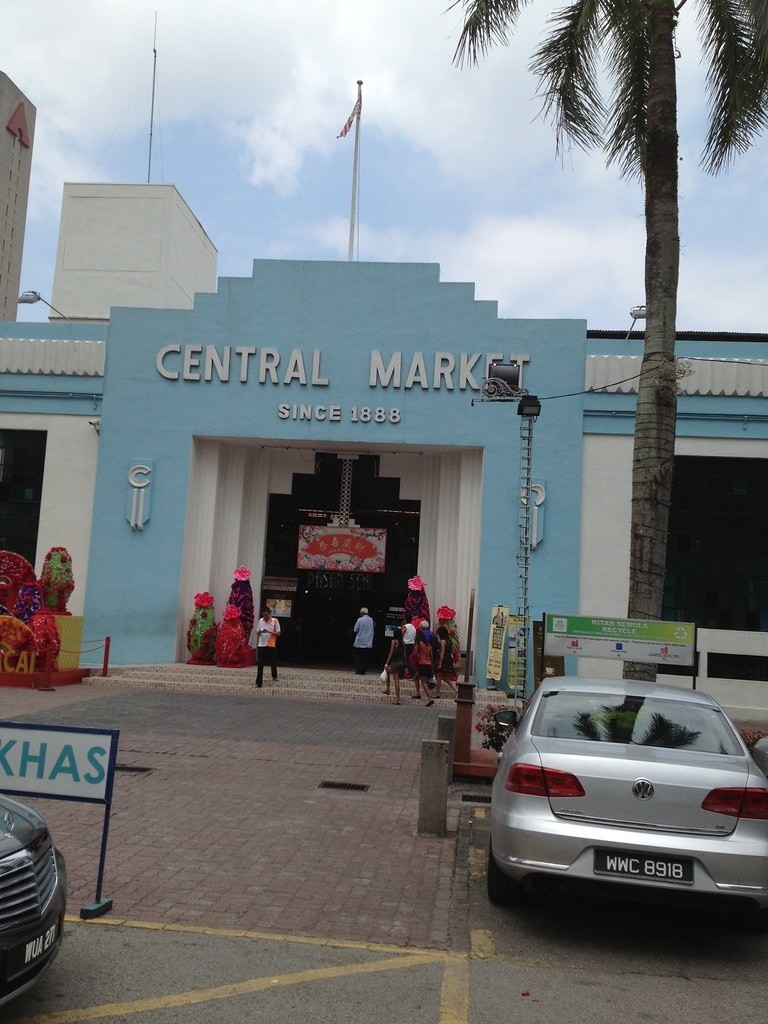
[335,92,361,140]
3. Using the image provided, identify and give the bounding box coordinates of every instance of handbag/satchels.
[427,679,437,690]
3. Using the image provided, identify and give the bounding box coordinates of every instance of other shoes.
[255,680,262,688]
[272,675,277,681]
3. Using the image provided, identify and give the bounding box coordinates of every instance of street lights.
[517,395,541,704]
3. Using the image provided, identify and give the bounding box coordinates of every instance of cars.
[0,793,67,1004]
[487,677,768,911]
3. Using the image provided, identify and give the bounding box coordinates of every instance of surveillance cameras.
[88,419,100,425]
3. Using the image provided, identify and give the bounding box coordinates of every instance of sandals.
[392,700,401,704]
[382,689,390,694]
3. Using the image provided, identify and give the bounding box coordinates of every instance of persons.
[352,607,375,674]
[256,607,280,688]
[382,628,407,704]
[401,612,458,706]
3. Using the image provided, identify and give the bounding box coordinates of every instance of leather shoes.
[411,694,422,699]
[432,695,440,699]
[425,700,434,707]
